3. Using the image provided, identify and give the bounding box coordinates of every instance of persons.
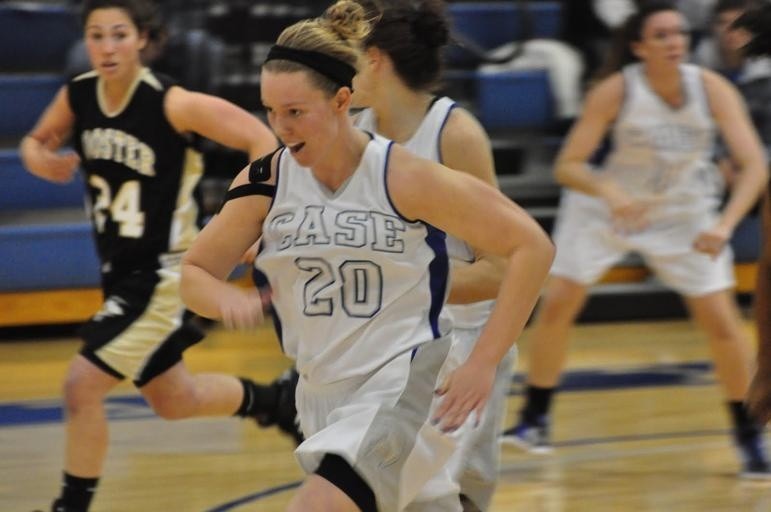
[16,2,306,511]
[741,194,771,421]
[724,12,770,151]
[498,10,771,483]
[177,2,556,510]
[327,2,510,510]
[682,0,768,63]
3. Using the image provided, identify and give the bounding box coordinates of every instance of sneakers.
[257,366,308,452]
[498,412,556,455]
[737,439,771,479]
